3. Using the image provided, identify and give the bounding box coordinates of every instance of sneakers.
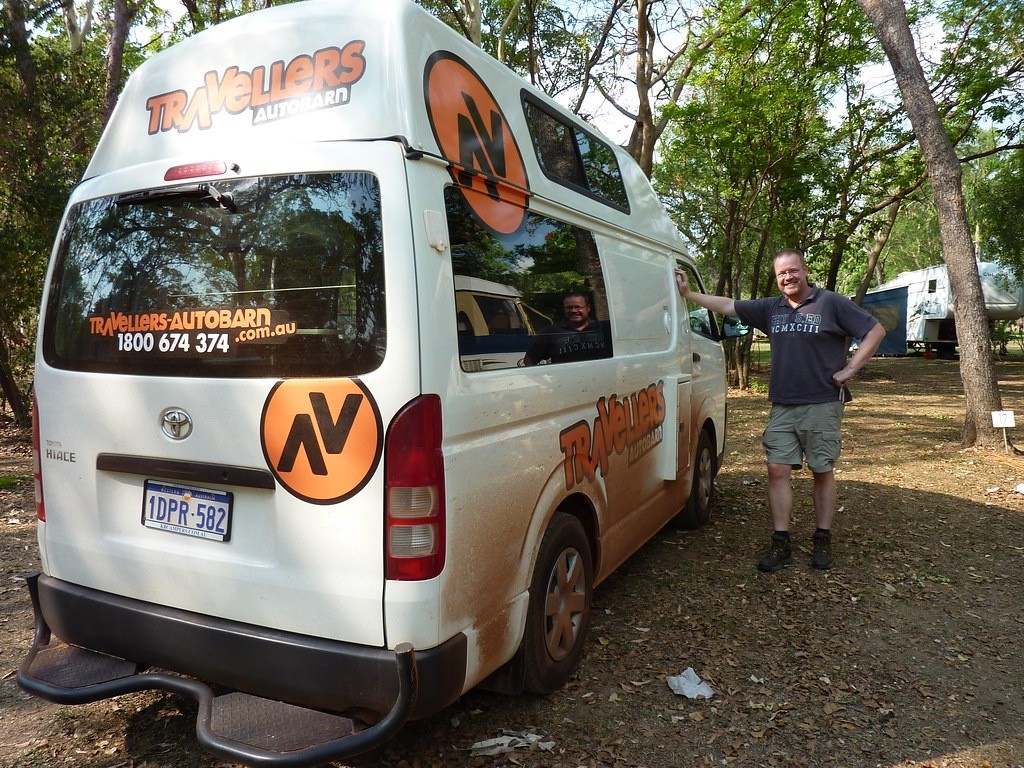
[756,537,792,570]
[812,533,834,568]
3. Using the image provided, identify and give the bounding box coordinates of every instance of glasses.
[776,265,803,277]
[564,303,591,310]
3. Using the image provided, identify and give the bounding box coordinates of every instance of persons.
[673,247,887,573]
[523,291,614,369]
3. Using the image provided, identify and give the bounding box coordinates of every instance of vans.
[454,274,543,372]
[14,0,751,766]
[846,257,1024,359]
